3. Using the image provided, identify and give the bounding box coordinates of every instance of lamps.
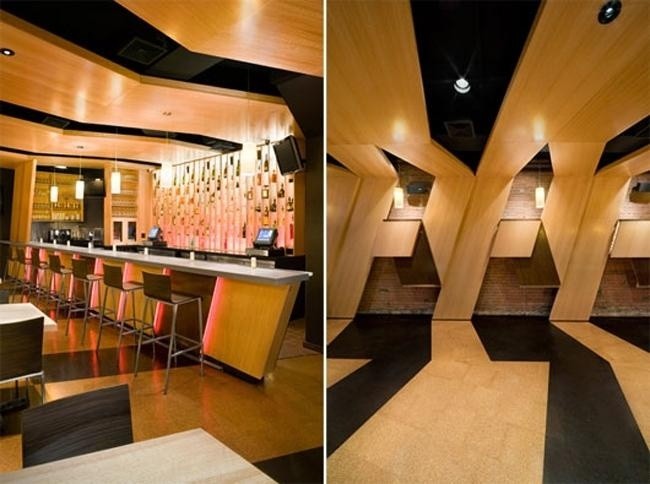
[453,76,470,95]
[48,164,59,204]
[239,101,256,176]
[110,151,121,195]
[159,112,173,189]
[74,146,86,201]
[393,157,404,210]
[534,155,545,209]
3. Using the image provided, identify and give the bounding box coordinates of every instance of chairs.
[20,384,134,468]
[1,316,45,439]
[0,242,204,397]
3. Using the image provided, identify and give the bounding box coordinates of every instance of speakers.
[637,182,650,193]
[406,181,426,193]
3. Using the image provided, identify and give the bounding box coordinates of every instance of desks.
[1,427,276,483]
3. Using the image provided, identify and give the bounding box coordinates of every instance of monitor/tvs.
[82,178,105,198]
[254,227,278,246]
[272,135,305,176]
[147,226,161,240]
